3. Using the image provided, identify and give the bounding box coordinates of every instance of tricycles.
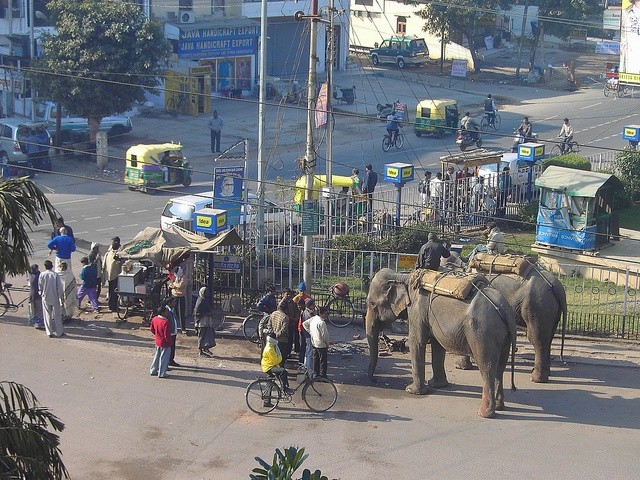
[603,69,635,98]
[124,141,193,195]
[333,84,357,105]
[291,175,368,226]
[414,99,460,138]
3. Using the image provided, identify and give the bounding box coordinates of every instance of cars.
[477,153,546,202]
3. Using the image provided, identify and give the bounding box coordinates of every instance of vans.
[35,100,132,146]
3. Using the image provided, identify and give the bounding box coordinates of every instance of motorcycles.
[510,128,538,153]
[455,124,484,151]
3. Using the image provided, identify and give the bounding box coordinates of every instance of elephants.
[439,250,568,383]
[366,268,520,419]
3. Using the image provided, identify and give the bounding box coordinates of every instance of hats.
[298,282,306,291]
[428,233,437,241]
[305,298,313,306]
[90,241,98,252]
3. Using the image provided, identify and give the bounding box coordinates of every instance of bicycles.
[242,308,270,347]
[382,124,404,152]
[244,362,337,415]
[549,136,581,160]
[0,282,29,317]
[480,105,501,132]
[166,89,189,116]
[315,284,356,328]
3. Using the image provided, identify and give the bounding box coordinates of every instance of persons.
[149,305,169,378]
[38,260,66,338]
[76,257,100,314]
[459,221,504,268]
[267,299,290,373]
[257,286,276,317]
[47,227,77,272]
[168,266,186,334]
[165,297,180,366]
[208,110,224,153]
[258,314,275,365]
[415,233,451,271]
[302,306,333,383]
[557,117,574,156]
[46,217,77,257]
[362,163,377,213]
[386,110,403,143]
[193,287,217,358]
[161,151,174,166]
[300,297,316,375]
[260,330,294,407]
[296,299,305,365]
[101,236,122,301]
[87,242,103,304]
[153,307,172,371]
[101,242,123,312]
[29,264,45,328]
[57,261,77,323]
[293,282,312,353]
[482,93,498,127]
[515,116,532,139]
[349,167,367,222]
[336,187,350,215]
[460,112,481,141]
[418,167,514,215]
[277,287,295,359]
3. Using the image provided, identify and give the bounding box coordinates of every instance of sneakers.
[160,374,168,378]
[94,308,99,313]
[263,402,275,407]
[282,387,294,394]
[151,372,158,375]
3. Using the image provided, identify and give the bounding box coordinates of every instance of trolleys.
[114,273,172,322]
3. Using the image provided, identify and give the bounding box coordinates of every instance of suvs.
[0,118,51,169]
[160,191,302,245]
[368,36,429,69]
